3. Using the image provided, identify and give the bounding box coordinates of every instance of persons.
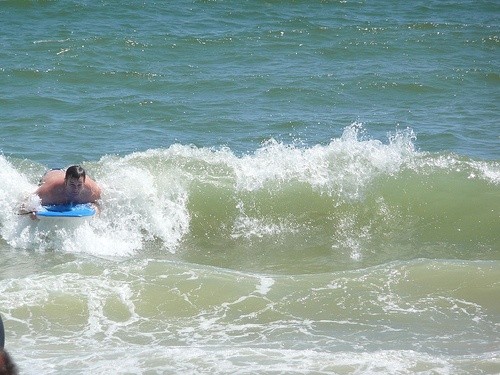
[16,165,103,223]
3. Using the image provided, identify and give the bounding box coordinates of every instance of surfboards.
[34,203,96,217]
